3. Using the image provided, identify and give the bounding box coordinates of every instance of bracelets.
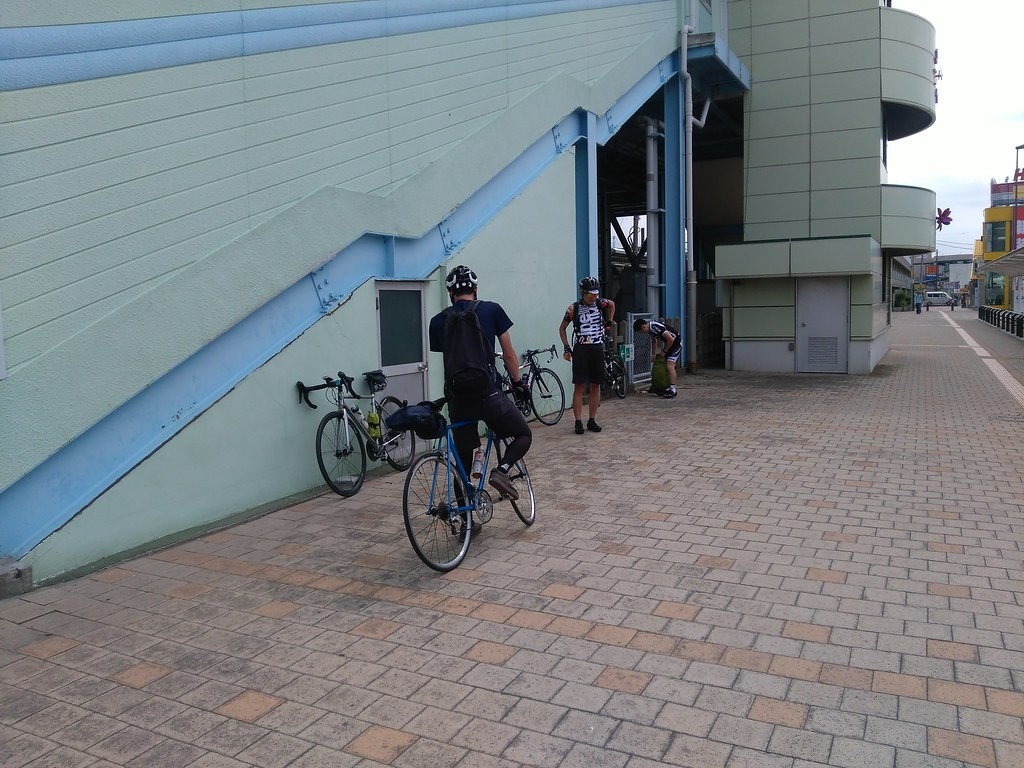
[663,349,667,353]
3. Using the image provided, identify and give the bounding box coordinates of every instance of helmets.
[578,276,599,294]
[445,265,477,288]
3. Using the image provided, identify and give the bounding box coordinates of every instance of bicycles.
[296,368,416,497]
[494,344,566,426]
[602,335,628,399]
[403,385,537,572]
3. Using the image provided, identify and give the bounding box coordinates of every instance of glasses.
[582,290,598,298]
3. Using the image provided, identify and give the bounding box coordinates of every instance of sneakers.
[489,468,519,500]
[460,523,482,543]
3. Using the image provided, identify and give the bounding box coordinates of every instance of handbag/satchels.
[384,404,448,439]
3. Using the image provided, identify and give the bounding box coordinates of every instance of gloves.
[513,380,531,402]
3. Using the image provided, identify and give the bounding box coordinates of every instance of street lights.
[1014,144,1024,249]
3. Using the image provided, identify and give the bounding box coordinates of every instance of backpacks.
[442,300,497,405]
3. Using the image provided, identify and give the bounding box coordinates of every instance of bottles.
[522,373,528,386]
[351,405,369,429]
[471,446,484,478]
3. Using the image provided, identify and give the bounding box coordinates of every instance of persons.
[429,265,532,543]
[560,276,615,435]
[915,293,922,314]
[634,319,682,398]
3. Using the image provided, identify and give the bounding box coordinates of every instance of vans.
[925,292,952,306]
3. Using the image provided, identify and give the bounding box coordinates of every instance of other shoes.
[587,421,602,432]
[575,423,584,434]
[663,388,677,398]
